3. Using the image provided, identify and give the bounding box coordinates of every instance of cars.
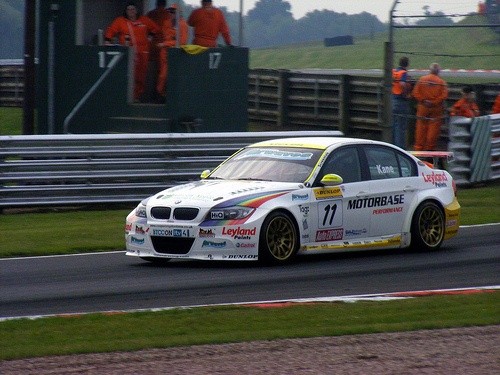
[125,138,462,267]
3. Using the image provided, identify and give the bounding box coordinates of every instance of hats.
[169,4,177,9]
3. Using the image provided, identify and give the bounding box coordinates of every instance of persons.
[391,56,418,149]
[147,0,190,102]
[187,0,233,47]
[491,93,500,115]
[105,4,161,104]
[450,86,480,118]
[412,63,449,151]
